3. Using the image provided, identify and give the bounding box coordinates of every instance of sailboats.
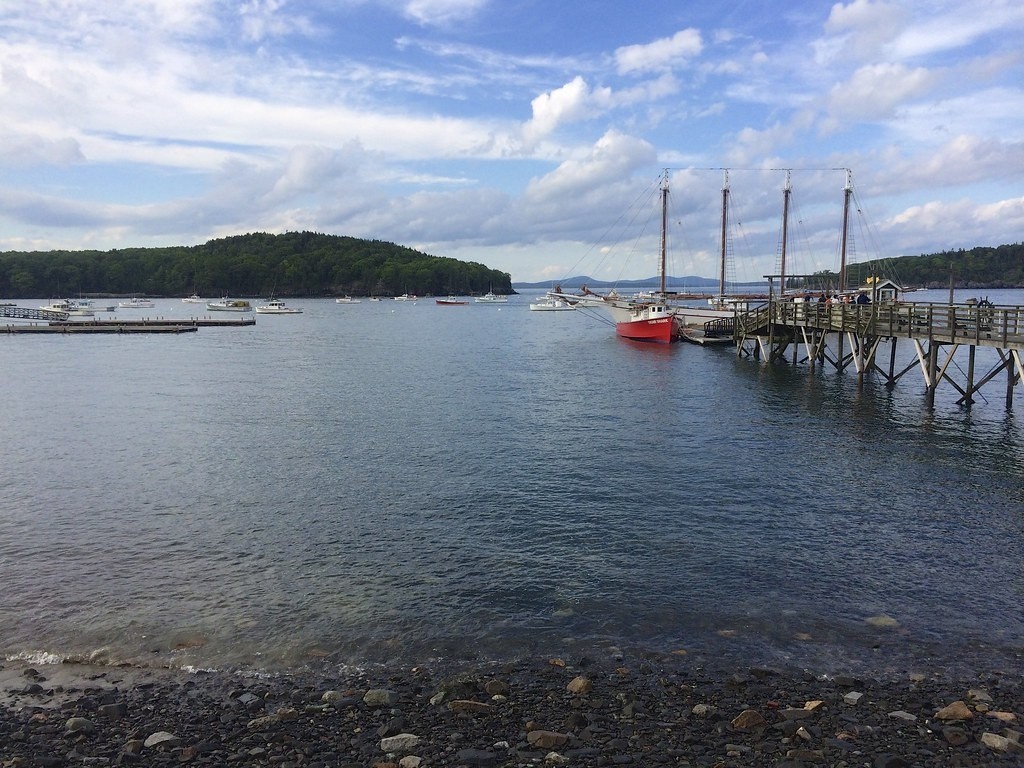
[547,167,918,329]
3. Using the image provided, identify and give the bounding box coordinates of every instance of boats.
[784,288,811,294]
[69,300,117,312]
[182,294,205,303]
[577,295,599,307]
[475,292,508,303]
[336,297,361,303]
[529,300,576,310]
[436,297,469,304]
[615,306,683,344]
[395,294,417,301]
[119,297,155,309]
[206,299,253,312]
[256,299,304,313]
[369,297,380,302]
[41,303,94,316]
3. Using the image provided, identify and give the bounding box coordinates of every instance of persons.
[805,293,872,306]
